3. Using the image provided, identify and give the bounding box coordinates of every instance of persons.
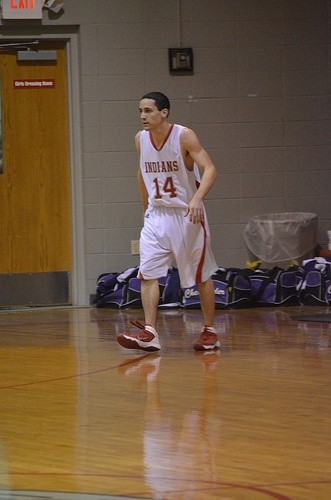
[117,350,222,500]
[117,91,221,352]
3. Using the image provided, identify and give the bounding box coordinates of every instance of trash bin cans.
[243,212,319,269]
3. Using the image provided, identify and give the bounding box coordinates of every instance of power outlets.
[131,240,139,255]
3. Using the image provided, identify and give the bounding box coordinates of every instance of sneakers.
[193,325,221,350]
[117,320,161,352]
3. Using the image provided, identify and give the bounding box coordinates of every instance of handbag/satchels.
[95,256,331,309]
